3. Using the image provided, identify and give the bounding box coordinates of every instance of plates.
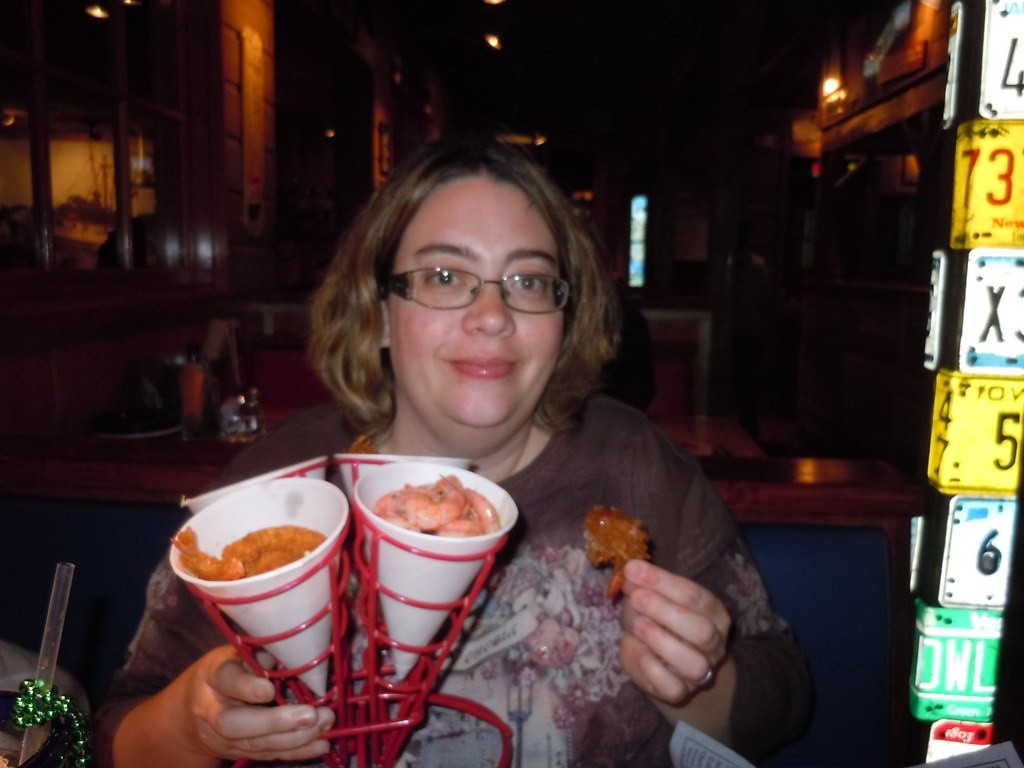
[87,407,184,440]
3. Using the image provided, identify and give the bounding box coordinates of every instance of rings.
[691,669,715,688]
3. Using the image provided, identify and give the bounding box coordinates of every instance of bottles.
[180,343,204,425]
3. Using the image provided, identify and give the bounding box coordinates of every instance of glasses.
[384,267,568,313]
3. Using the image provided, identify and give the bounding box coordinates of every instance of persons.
[84,138,816,766]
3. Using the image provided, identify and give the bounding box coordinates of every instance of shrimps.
[370,475,499,539]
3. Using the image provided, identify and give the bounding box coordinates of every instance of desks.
[81,404,775,480]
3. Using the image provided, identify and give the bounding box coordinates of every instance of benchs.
[0,302,928,767]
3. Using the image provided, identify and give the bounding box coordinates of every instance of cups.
[0,689,92,768]
[220,390,259,442]
